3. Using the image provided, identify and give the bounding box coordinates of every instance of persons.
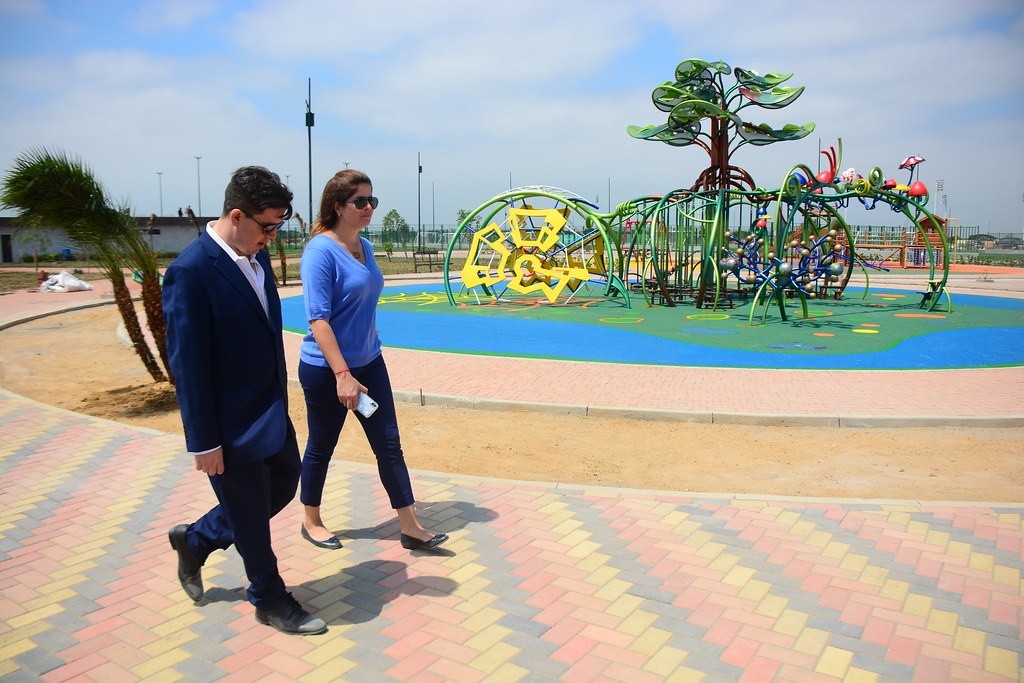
[178,205,191,217]
[298,170,449,550]
[161,165,328,636]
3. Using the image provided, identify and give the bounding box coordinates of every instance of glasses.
[229,206,285,234]
[338,197,379,209]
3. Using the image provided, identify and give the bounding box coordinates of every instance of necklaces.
[339,235,360,259]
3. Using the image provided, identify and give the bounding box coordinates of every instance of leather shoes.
[301,523,341,548]
[255,594,327,635]
[400,534,449,550]
[168,523,203,602]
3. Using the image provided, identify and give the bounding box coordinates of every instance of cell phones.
[356,392,379,418]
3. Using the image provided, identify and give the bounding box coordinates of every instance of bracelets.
[335,370,350,380]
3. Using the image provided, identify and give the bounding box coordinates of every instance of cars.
[969,234,994,240]
[995,236,1024,247]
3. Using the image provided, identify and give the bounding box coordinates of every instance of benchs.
[413,250,453,273]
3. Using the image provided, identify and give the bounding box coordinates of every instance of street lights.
[285,175,293,247]
[156,171,164,216]
[194,154,203,217]
